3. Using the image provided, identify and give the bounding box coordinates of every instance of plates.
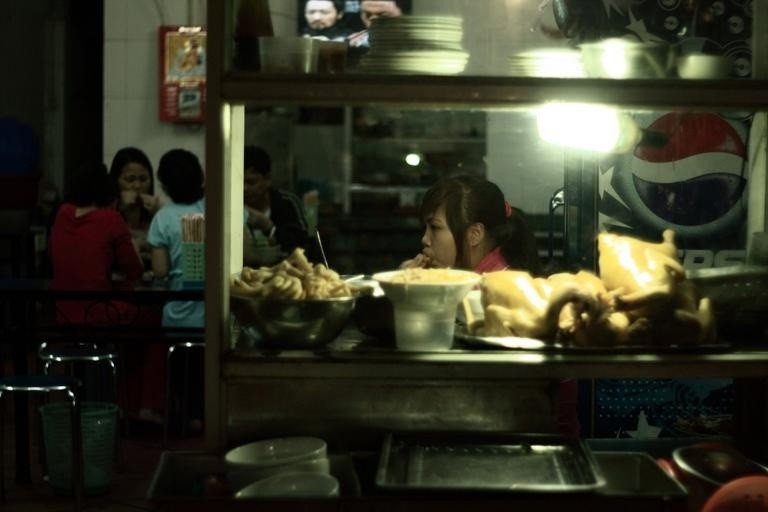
[507,47,588,80]
[451,325,547,351]
[359,13,470,79]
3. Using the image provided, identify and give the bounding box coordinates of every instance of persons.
[105,146,162,271]
[241,144,324,278]
[397,172,543,277]
[49,161,147,436]
[145,148,208,429]
[346,0,412,48]
[304,0,350,41]
[292,178,348,279]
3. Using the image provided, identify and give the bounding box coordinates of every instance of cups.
[391,303,459,351]
[256,38,320,76]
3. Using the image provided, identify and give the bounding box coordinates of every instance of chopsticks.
[179,215,206,243]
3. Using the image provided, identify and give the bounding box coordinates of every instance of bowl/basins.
[225,436,327,466]
[229,293,363,350]
[677,55,734,79]
[227,459,330,491]
[234,471,340,497]
[371,270,482,311]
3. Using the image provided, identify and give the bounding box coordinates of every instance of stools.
[39,338,127,475]
[163,332,206,432]
[0,372,84,511]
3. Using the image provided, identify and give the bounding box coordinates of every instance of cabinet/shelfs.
[204,1,768,512]
